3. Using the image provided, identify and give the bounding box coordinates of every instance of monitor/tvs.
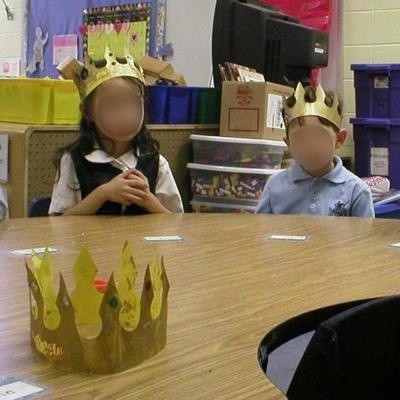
[212,0,329,87]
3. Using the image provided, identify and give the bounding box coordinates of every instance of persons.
[23,25,49,75]
[254,86,376,220]
[49,55,185,216]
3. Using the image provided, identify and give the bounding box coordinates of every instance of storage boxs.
[350,61,399,219]
[0,76,220,126]
[188,81,298,213]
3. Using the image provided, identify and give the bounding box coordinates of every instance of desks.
[0,125,220,217]
